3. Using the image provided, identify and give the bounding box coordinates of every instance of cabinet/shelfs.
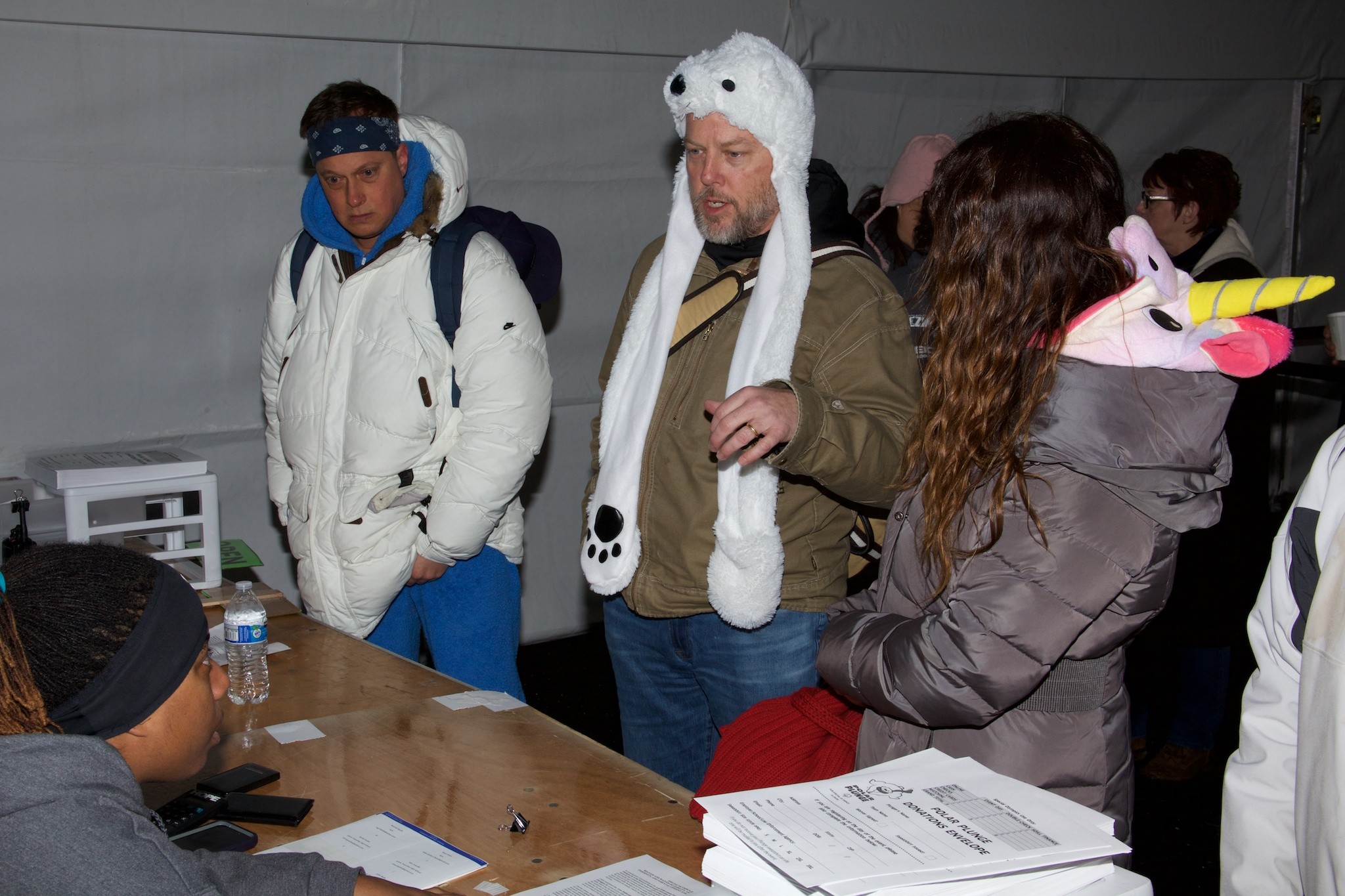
[35,470,223,590]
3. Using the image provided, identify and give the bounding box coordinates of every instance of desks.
[123,532,710,896]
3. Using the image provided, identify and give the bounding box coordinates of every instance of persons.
[817,112,1336,863]
[582,31,923,793]
[1219,423,1344,896]
[1138,146,1278,432]
[0,542,461,896]
[805,128,954,596]
[1322,323,1338,364]
[261,83,563,708]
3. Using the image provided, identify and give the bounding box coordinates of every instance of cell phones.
[166,820,257,853]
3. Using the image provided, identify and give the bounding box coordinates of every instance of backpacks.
[289,204,563,354]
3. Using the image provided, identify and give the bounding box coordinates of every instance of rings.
[747,422,757,436]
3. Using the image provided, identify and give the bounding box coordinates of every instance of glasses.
[1139,190,1175,210]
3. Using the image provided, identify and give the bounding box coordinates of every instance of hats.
[577,30,816,629]
[866,133,956,271]
[0,540,210,741]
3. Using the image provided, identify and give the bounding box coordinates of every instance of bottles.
[224,581,270,706]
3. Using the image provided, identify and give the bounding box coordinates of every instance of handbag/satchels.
[689,687,864,827]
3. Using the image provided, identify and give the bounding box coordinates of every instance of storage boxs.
[0,478,147,564]
